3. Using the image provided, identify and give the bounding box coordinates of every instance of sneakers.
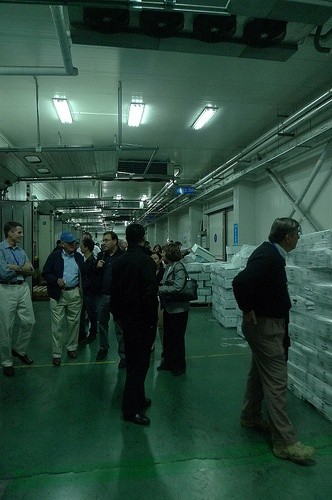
[241,416,272,430]
[274,440,315,460]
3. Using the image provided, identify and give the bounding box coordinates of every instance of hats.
[60,232,76,242]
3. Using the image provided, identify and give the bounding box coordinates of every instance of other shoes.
[126,413,150,425]
[157,362,169,370]
[117,358,126,369]
[79,333,86,337]
[96,351,107,360]
[143,398,151,408]
[174,368,185,375]
[88,333,96,340]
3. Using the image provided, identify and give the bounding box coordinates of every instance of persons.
[145,241,166,268]
[41,232,83,365]
[53,232,101,256]
[157,244,190,376]
[232,217,313,460]
[111,224,158,426]
[81,238,97,344]
[95,232,126,369]
[149,252,163,362]
[0,222,35,377]
[118,239,127,250]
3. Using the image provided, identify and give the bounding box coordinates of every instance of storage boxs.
[181,244,258,339]
[287,230,332,422]
[32,286,48,297]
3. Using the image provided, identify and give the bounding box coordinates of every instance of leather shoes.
[68,350,77,358]
[12,349,33,364]
[3,366,14,376]
[53,358,61,365]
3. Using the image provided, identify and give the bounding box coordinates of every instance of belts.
[0,280,23,285]
[63,284,78,290]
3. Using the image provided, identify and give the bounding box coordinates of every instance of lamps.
[190,107,219,131]
[52,98,73,124]
[128,103,145,127]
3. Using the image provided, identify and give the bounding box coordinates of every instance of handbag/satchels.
[162,263,198,303]
